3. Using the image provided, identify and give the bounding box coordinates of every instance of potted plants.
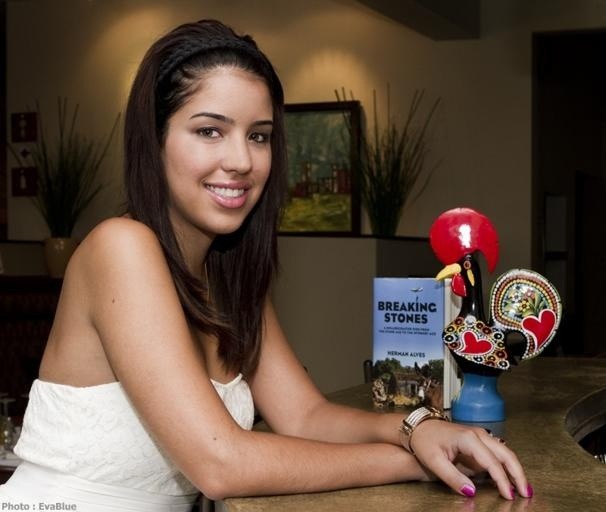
[0,94,122,281]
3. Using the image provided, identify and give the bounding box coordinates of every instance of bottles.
[1,399,23,448]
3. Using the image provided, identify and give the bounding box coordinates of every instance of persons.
[1,20,533,511]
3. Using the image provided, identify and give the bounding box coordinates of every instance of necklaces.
[203,259,212,308]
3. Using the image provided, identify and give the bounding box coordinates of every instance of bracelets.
[399,403,451,455]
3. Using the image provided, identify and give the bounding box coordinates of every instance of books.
[371,277,462,408]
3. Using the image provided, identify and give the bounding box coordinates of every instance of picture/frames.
[274,101,362,238]
